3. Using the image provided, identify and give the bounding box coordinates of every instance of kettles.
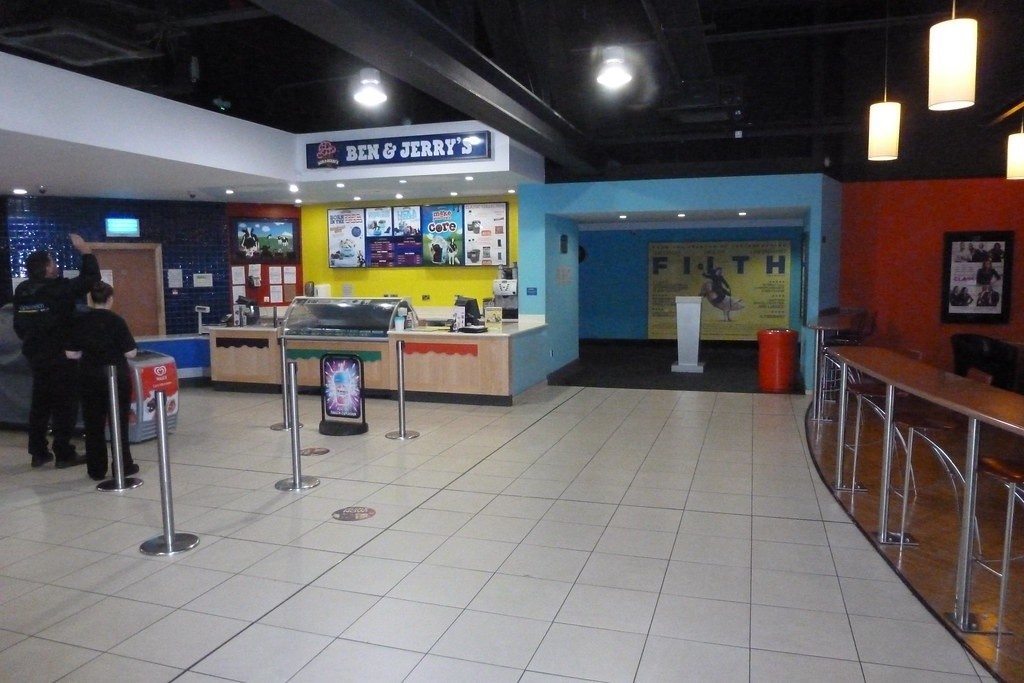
[305,281,314,297]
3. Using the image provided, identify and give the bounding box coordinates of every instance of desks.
[822,344,1023,632]
[810,311,861,422]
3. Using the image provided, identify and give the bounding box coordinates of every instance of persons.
[976,260,1001,284]
[13,234,101,469]
[65,280,139,480]
[955,242,1005,262]
[977,286,999,307]
[950,286,973,306]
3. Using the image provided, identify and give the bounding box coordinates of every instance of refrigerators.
[106,348,179,442]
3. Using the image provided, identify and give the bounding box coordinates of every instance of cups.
[334,372,351,405]
[394,316,405,331]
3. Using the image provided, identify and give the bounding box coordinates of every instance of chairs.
[951,331,1024,426]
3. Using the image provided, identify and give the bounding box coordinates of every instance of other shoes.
[92,474,104,480]
[32,453,53,466]
[56,453,87,467]
[113,464,139,477]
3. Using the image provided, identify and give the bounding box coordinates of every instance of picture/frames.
[940,229,1015,324]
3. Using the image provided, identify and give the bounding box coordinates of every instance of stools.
[827,335,861,400]
[842,381,1023,646]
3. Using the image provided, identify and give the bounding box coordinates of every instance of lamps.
[867,0,1024,181]
[352,65,387,107]
[596,44,633,92]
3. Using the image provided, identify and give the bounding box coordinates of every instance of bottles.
[512,261,518,279]
[239,313,247,328]
[406,316,412,328]
